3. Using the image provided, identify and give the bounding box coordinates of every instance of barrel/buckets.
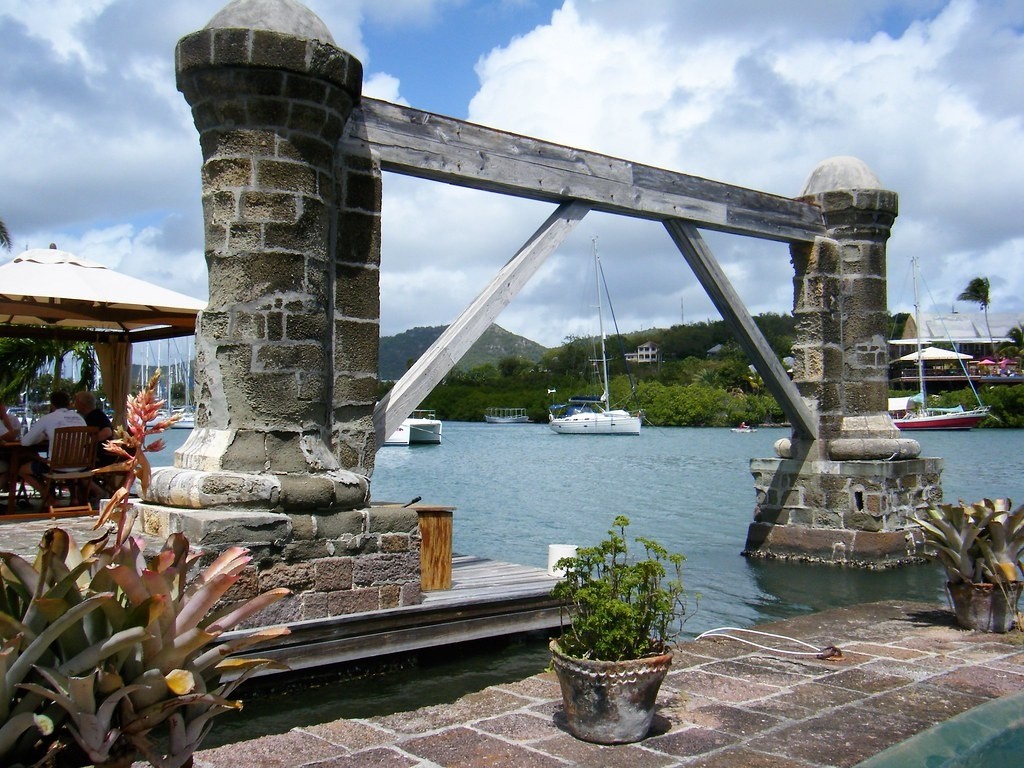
[547,544,578,578]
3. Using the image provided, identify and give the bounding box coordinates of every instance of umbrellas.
[997,359,1018,365]
[977,358,996,377]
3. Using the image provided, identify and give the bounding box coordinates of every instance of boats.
[484,407,529,423]
[383,410,442,446]
[730,428,757,433]
[548,404,596,421]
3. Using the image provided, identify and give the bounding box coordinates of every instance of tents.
[0,248,210,433]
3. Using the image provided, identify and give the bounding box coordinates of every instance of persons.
[0,397,22,505]
[19,390,91,514]
[739,421,749,429]
[73,391,115,509]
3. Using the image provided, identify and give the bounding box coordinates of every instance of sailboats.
[549,239,645,435]
[128,336,194,429]
[888,256,991,430]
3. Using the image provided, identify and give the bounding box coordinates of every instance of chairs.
[92,426,136,509]
[39,426,99,520]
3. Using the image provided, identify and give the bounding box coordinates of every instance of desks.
[370,500,458,591]
[0,440,49,514]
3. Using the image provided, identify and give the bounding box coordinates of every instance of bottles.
[20,415,28,437]
[30,415,36,430]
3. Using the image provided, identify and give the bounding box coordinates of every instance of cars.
[6,408,40,430]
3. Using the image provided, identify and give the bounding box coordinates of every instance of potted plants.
[543,515,700,745]
[899,497,1024,634]
